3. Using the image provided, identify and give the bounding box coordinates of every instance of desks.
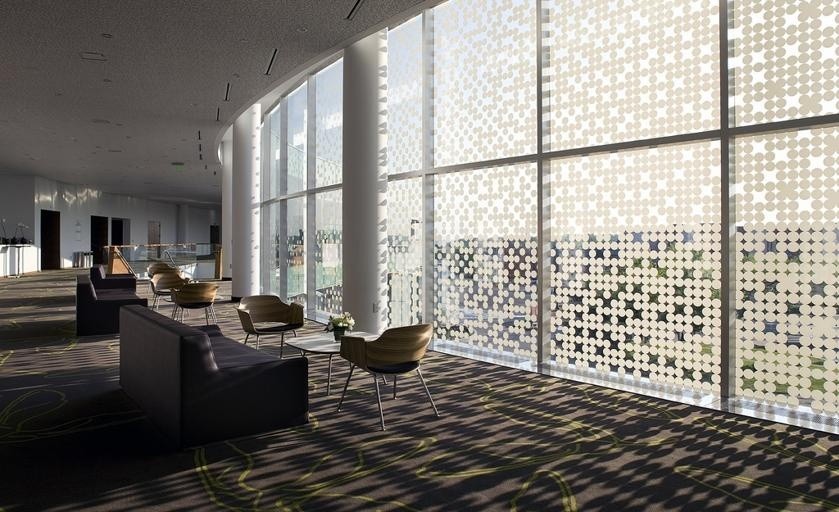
[6,245,32,280]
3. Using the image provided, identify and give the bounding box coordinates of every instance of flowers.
[324,312,355,333]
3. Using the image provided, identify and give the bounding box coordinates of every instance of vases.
[334,327,346,341]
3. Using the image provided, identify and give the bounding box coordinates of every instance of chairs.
[147,260,220,326]
[336,323,440,430]
[232,295,304,360]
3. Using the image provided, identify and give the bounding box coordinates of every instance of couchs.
[120,304,308,447]
[77,264,145,335]
